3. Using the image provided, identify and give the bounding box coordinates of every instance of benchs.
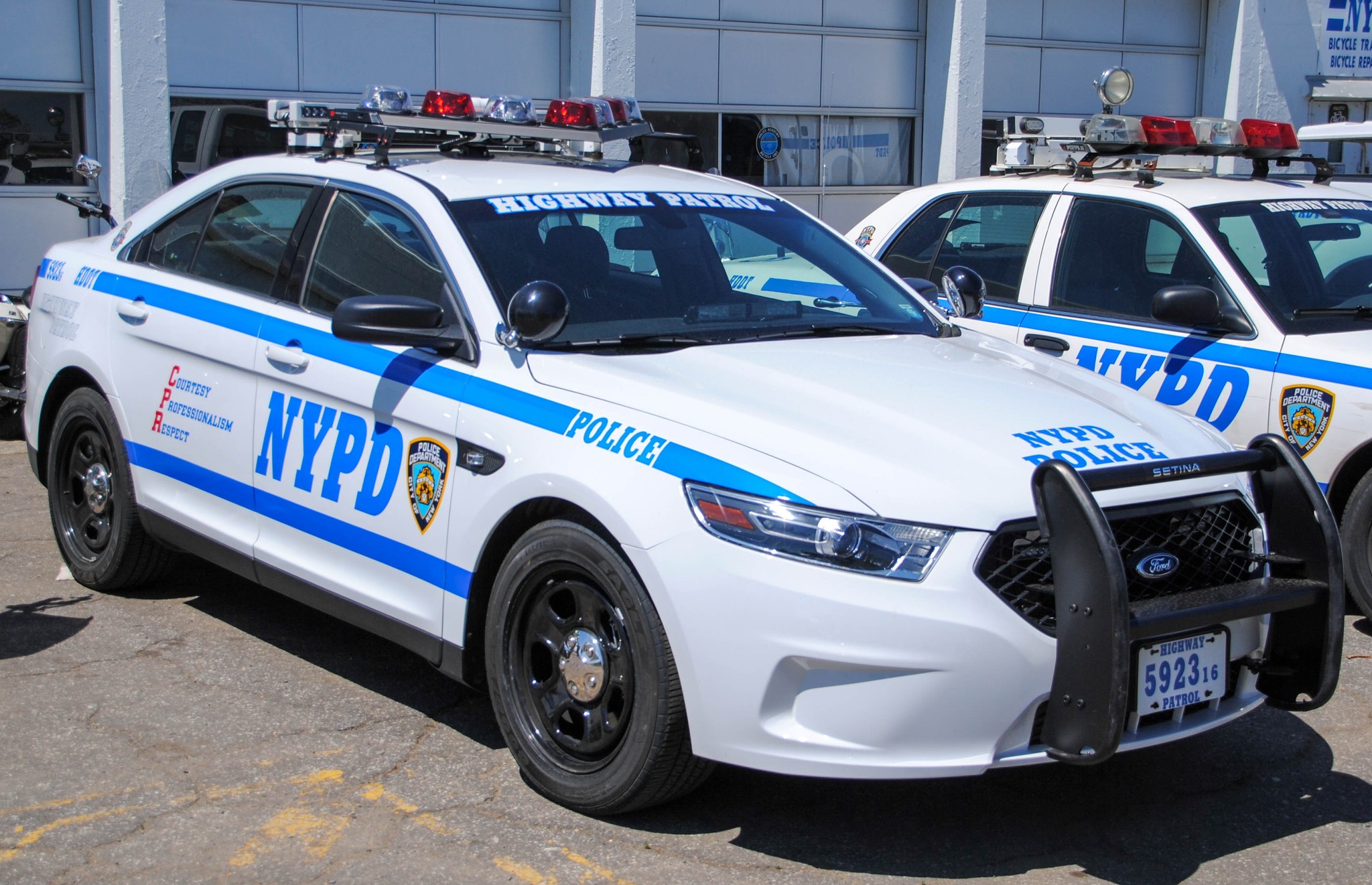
[918,254,1025,288]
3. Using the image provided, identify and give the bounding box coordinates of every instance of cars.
[21,87,1347,820]
[651,64,1372,631]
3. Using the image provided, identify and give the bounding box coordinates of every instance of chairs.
[1179,233,1229,280]
[544,225,609,284]
